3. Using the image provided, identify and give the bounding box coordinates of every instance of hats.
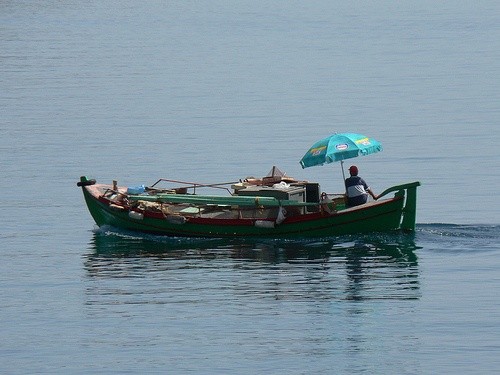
[348,166,358,173]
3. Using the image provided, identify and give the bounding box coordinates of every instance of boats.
[77,165,419,242]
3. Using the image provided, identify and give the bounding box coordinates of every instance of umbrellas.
[299,132,383,208]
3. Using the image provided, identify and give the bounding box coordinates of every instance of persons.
[344,166,375,207]
